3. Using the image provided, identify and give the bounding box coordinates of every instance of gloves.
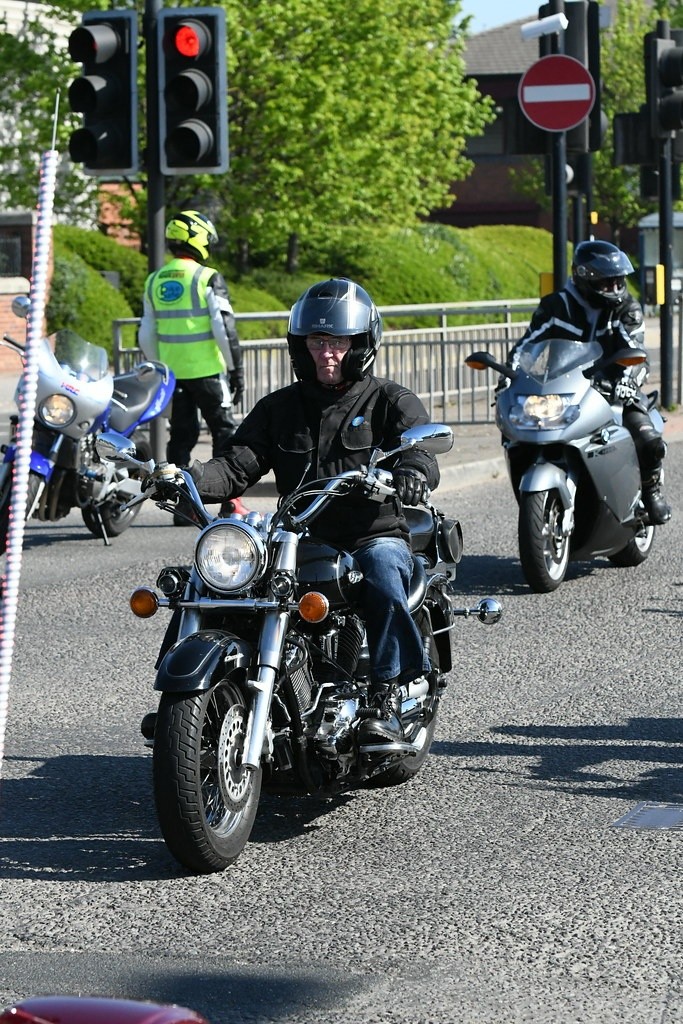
[614,377,638,407]
[230,371,246,405]
[391,466,429,506]
[140,464,186,504]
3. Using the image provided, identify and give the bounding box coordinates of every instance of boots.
[640,483,672,526]
[140,713,157,741]
[359,682,404,742]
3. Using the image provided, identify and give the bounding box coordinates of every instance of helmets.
[571,240,635,310]
[286,277,385,391]
[164,209,219,261]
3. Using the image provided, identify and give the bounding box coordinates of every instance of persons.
[495,240,672,525]
[138,210,252,527]
[142,279,440,741]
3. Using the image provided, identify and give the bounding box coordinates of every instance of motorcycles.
[95,420,504,874]
[1,295,178,551]
[461,311,664,592]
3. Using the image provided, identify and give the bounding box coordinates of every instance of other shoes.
[174,506,202,528]
[221,498,255,518]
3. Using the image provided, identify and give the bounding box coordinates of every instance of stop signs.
[519,54,595,134]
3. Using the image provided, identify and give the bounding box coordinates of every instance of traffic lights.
[64,8,136,179]
[153,5,231,177]
[649,37,683,142]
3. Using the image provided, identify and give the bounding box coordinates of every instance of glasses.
[306,337,353,351]
[601,277,625,291]
